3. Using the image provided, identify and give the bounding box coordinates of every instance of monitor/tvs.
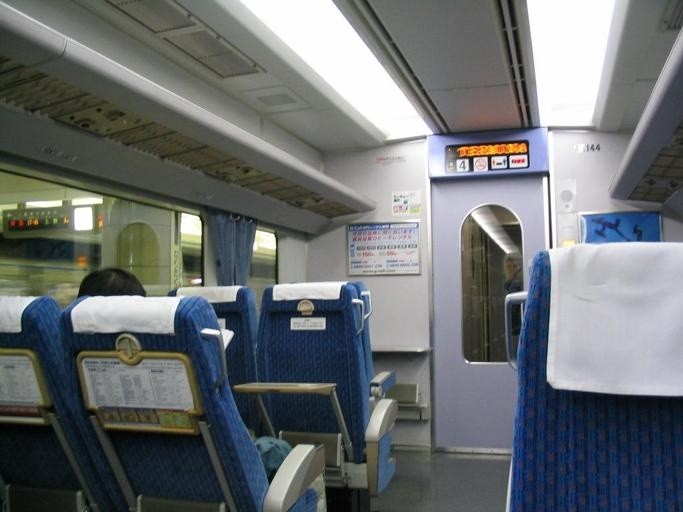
[73,205,95,233]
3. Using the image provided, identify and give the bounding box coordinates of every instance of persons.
[498,250,523,346]
[69,271,144,295]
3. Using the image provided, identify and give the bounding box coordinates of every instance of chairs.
[499,243,683,509]
